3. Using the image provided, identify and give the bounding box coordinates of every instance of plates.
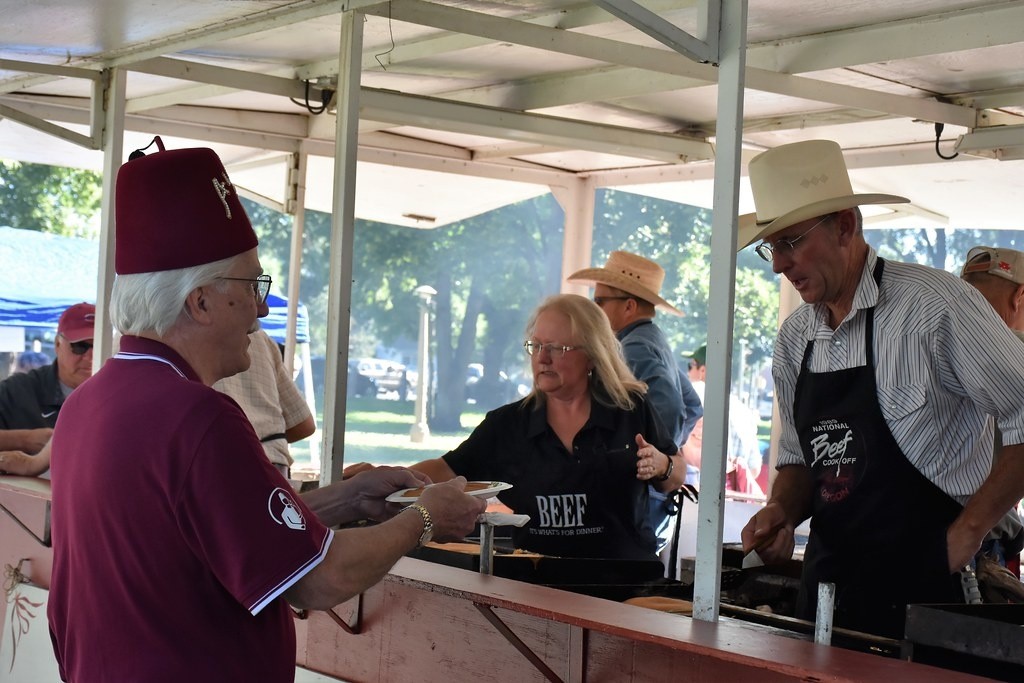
[385,480,515,505]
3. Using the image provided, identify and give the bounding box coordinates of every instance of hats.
[115,136,261,274]
[682,344,706,366]
[58,302,95,343]
[567,251,684,317]
[960,246,1024,285]
[734,140,911,252]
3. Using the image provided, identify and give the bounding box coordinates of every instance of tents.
[0,225,321,473]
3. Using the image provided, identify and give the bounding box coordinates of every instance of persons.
[340,293,687,607]
[47,137,489,683]
[565,251,704,547]
[942,245,1024,588]
[711,139,1024,666]
[2,303,317,478]
[670,343,752,498]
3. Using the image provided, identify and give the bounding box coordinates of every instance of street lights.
[409,285,437,442]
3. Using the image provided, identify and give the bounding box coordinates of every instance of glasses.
[523,340,584,358]
[755,212,839,262]
[58,332,93,354]
[688,363,698,371]
[595,296,639,308]
[214,274,272,305]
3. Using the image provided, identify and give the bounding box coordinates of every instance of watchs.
[660,453,675,484]
[399,504,434,549]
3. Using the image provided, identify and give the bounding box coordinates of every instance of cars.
[296,356,534,405]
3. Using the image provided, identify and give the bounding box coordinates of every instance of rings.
[477,514,486,522]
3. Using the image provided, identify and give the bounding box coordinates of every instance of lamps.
[953,124,1024,161]
